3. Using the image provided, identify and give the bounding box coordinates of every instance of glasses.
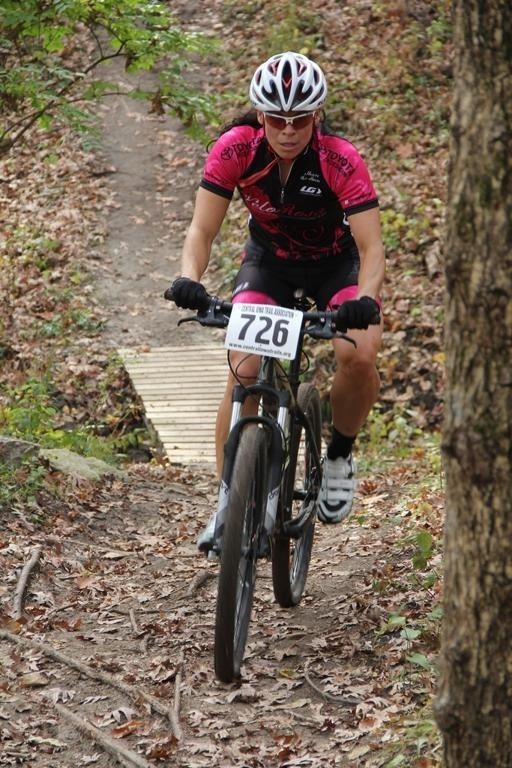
[261,113,315,130]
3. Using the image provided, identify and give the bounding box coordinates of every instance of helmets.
[249,51,329,113]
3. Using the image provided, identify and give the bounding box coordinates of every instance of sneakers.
[317,453,356,523]
[198,512,221,558]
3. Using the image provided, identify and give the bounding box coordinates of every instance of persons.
[163,50,390,556]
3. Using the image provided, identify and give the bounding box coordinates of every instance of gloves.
[171,275,208,310]
[334,293,381,333]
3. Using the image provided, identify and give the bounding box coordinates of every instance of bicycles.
[164,289,379,687]
